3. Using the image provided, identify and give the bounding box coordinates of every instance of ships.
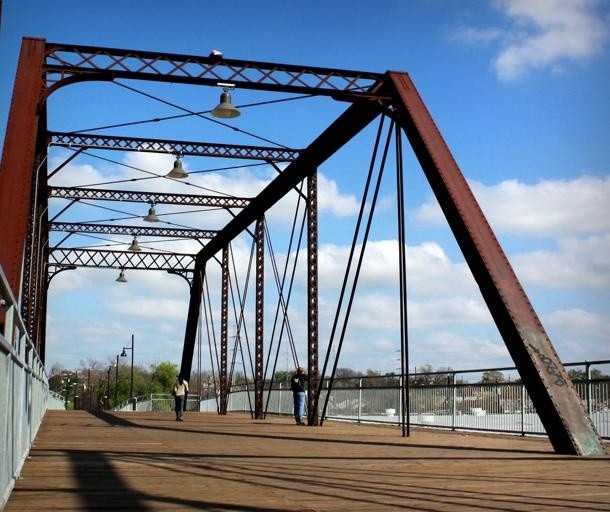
[436,410,462,417]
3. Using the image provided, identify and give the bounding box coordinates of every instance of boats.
[421,412,435,423]
[474,411,488,416]
[404,413,418,424]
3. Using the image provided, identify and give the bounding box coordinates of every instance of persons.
[173,373,189,422]
[290,367,308,425]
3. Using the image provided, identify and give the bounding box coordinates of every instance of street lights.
[121,334,135,404]
[60,354,120,410]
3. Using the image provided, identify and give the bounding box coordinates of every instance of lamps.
[143,203,160,222]
[210,85,241,119]
[165,150,190,179]
[115,268,128,282]
[127,235,141,251]
